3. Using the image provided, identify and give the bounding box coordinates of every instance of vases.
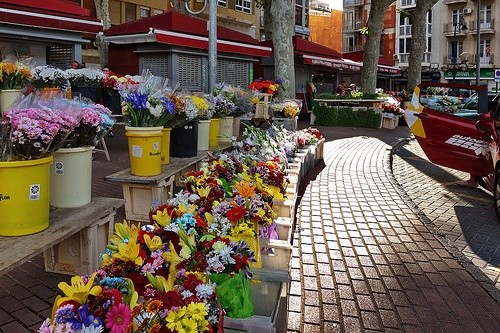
[125,125,163,134]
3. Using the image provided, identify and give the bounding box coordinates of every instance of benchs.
[0,197,125,277]
[104,140,237,222]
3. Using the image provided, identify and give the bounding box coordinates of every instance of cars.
[402,83,500,218]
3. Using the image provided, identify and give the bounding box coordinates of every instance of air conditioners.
[463,8,472,14]
[392,54,399,60]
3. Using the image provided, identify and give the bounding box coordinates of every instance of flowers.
[0,61,322,333]
[350,87,462,115]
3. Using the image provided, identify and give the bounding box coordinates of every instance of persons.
[337,81,356,95]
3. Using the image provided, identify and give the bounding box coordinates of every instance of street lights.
[460,0,481,87]
